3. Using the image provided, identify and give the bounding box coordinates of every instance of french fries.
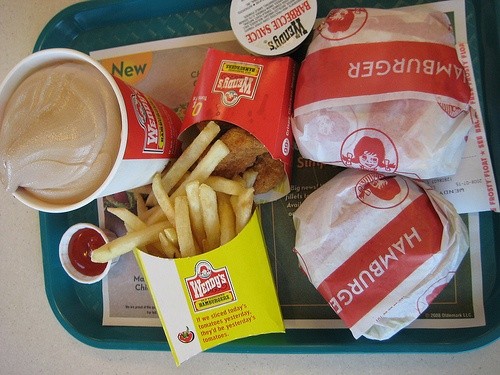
[91,120,258,265]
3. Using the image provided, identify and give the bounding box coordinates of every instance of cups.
[0,48,182,213]
[59,223,120,284]
